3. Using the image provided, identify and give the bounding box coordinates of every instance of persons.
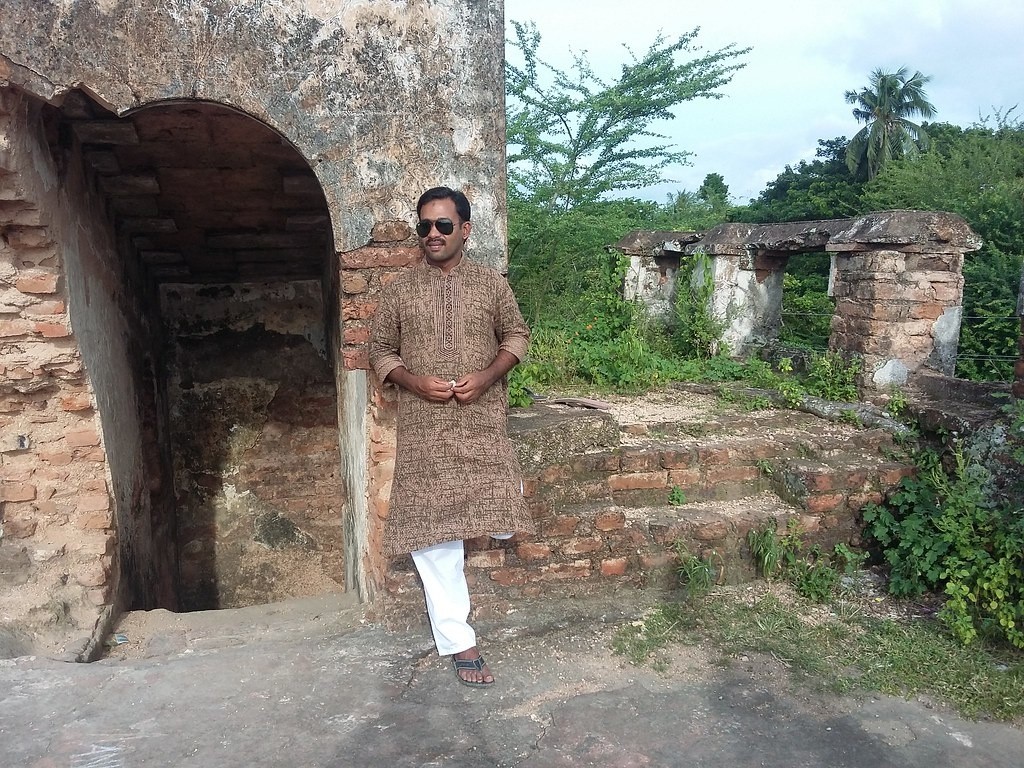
[369,186,538,690]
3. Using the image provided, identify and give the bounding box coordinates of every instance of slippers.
[451,652,494,688]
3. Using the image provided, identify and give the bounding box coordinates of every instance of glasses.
[416,218,463,238]
